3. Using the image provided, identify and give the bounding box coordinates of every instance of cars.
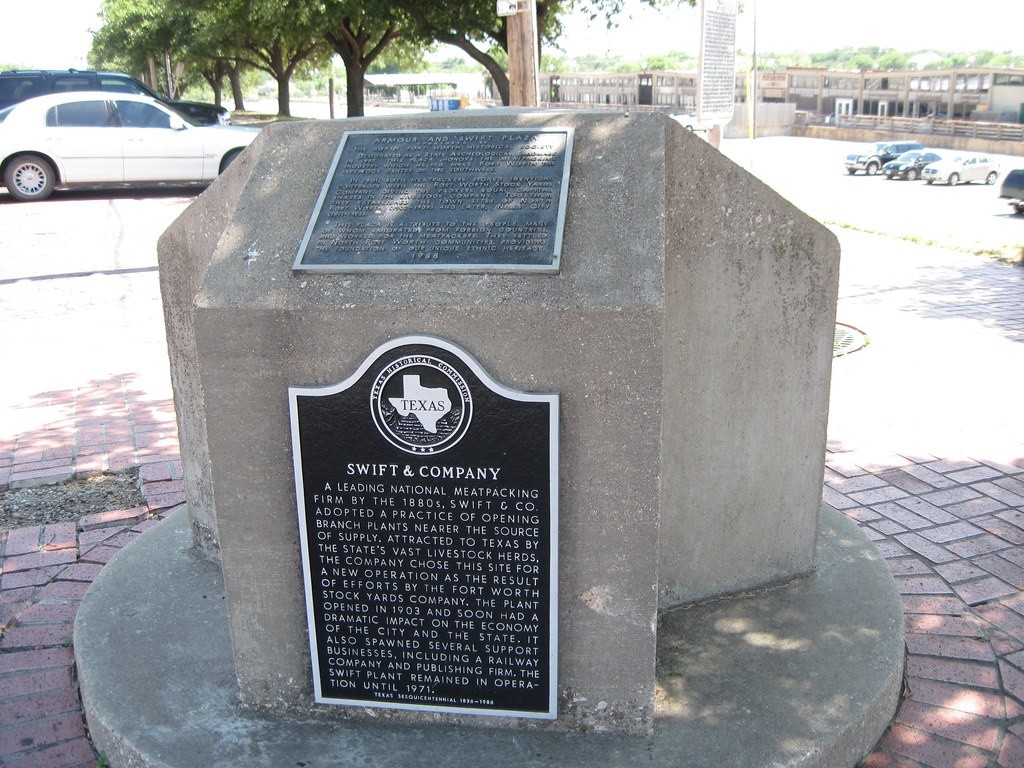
[927,113,947,120]
[998,168,1024,214]
[921,153,1000,185]
[0,90,266,202]
[883,148,943,181]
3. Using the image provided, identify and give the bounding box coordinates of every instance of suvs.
[0,66,229,124]
[844,141,924,176]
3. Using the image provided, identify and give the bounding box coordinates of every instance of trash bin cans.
[429,97,460,112]
